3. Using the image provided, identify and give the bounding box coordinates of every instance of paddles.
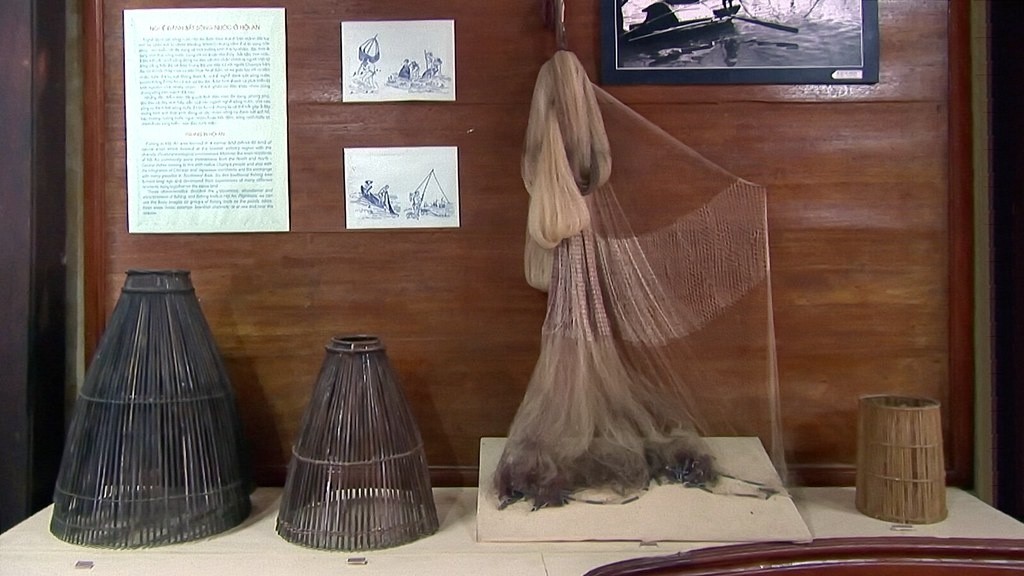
[731,15,798,33]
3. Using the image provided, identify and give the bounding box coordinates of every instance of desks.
[0,486,1024,576]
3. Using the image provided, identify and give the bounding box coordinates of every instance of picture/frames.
[598,0,880,85]
